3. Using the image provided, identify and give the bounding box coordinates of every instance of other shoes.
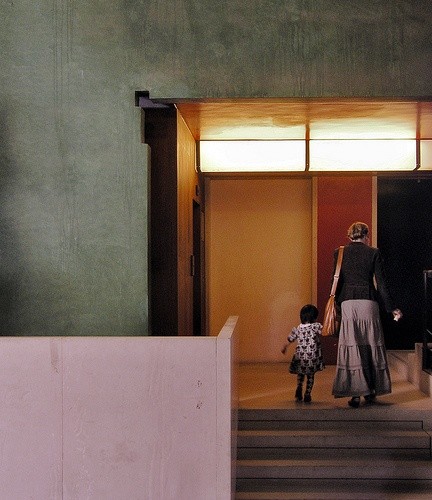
[304,395,311,402]
[365,395,375,401]
[348,399,360,408]
[295,391,303,400]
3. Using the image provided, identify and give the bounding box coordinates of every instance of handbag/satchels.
[321,296,341,337]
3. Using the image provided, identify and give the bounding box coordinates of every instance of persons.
[325,222,403,409]
[280,304,326,403]
